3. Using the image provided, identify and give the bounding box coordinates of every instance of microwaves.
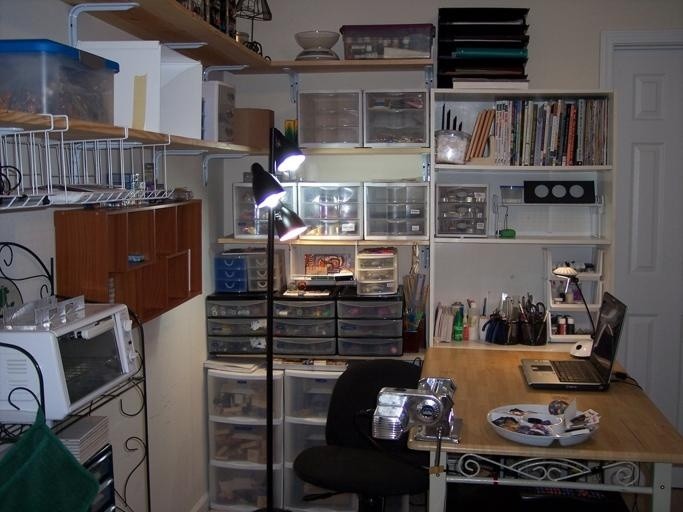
[1,300,141,421]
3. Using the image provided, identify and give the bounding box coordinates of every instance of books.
[495,93,613,169]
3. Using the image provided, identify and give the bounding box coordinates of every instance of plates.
[487,403,601,448]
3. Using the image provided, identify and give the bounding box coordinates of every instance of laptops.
[521,290,629,391]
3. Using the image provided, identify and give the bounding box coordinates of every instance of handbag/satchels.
[0,407,100,512]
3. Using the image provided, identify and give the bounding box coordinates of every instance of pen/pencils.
[518,301,532,345]
[536,309,549,343]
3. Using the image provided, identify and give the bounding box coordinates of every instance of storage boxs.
[435,183,489,238]
[363,90,429,149]
[297,182,363,240]
[363,181,430,240]
[297,91,363,149]
[343,23,435,58]
[232,183,298,238]
[0,40,119,125]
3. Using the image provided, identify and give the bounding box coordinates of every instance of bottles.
[558,318,565,335]
[467,303,479,341]
[566,318,574,334]
[453,310,463,341]
[451,302,465,338]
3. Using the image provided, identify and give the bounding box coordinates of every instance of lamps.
[251,129,306,512]
[553,268,595,358]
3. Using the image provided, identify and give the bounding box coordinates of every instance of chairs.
[293,357,433,512]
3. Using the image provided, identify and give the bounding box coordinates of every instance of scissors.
[531,303,546,315]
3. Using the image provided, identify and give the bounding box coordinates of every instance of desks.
[408,346,683,512]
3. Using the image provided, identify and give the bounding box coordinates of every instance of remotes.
[519,486,609,502]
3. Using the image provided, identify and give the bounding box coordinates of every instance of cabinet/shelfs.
[429,88,618,355]
[207,369,282,511]
[285,367,361,512]
[55,199,202,329]
